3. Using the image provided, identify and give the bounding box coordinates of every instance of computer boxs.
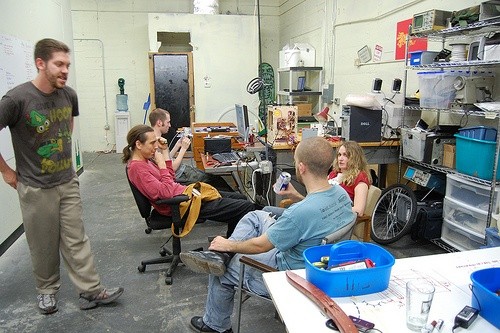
[341,105,382,142]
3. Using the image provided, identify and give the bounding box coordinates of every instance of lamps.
[313,108,338,136]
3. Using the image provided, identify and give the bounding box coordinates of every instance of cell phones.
[348,315,375,332]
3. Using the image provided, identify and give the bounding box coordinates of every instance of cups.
[405,279,434,331]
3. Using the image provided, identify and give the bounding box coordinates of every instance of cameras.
[184,134,194,139]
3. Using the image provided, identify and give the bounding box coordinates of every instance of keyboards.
[212,152,240,162]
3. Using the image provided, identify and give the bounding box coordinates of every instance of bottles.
[116,94,128,111]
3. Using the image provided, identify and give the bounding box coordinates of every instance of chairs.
[236,213,356,333]
[124,166,205,285]
[280,184,380,241]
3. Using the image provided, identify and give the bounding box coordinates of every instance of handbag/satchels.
[171,182,222,238]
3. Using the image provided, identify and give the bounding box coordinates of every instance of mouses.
[220,162,232,166]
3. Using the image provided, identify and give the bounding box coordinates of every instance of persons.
[123,108,254,239]
[179,135,357,332]
[0,38,124,313]
[327,141,372,217]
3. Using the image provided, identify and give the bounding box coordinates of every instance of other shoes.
[189,316,233,333]
[179,251,229,276]
[79,287,124,310]
[37,294,58,314]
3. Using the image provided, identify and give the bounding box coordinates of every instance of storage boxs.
[302,71,500,331]
[265,104,299,148]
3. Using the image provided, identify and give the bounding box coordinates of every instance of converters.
[454,305,479,329]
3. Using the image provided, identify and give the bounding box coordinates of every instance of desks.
[263,247,500,332]
[260,135,400,208]
[202,153,258,204]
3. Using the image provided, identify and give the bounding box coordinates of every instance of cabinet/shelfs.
[275,66,320,123]
[398,0,500,255]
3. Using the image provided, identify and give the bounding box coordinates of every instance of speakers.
[393,79,402,91]
[374,78,382,91]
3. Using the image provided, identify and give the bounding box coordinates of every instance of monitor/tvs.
[235,103,249,139]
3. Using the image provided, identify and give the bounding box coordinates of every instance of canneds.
[273,172,291,194]
